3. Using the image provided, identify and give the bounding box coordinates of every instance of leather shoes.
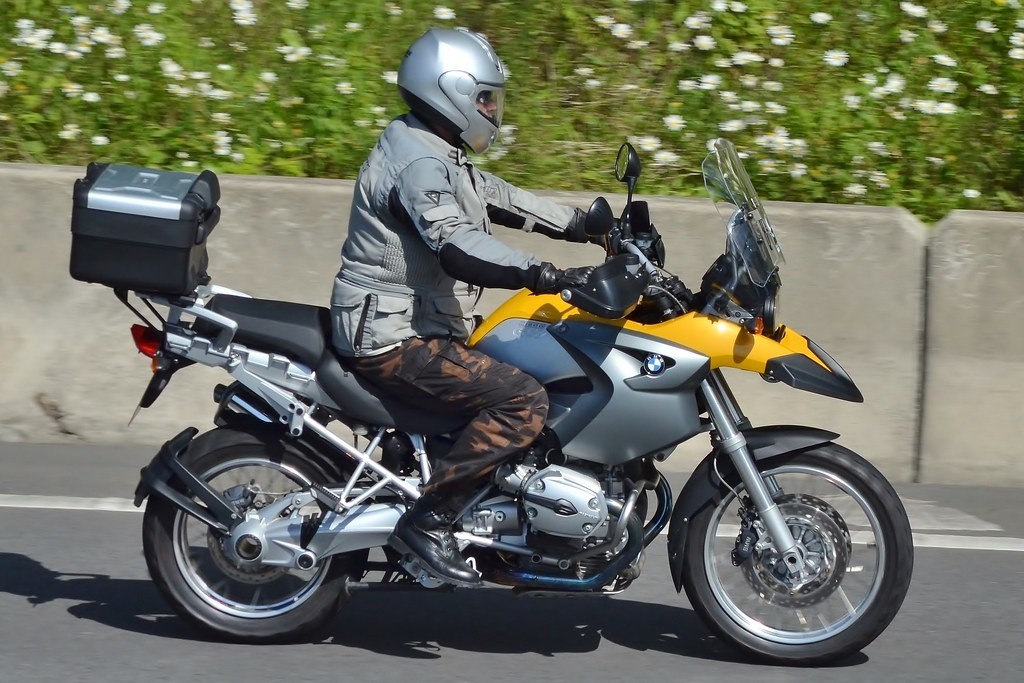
[388,497,481,589]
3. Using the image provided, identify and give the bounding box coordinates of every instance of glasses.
[477,92,490,104]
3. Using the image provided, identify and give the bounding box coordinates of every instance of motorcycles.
[70,137,914,660]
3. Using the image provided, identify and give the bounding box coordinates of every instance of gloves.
[536,262,595,294]
[567,208,620,248]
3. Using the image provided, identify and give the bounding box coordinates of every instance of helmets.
[397,27,505,154]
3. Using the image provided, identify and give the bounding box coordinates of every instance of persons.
[328,25,624,585]
